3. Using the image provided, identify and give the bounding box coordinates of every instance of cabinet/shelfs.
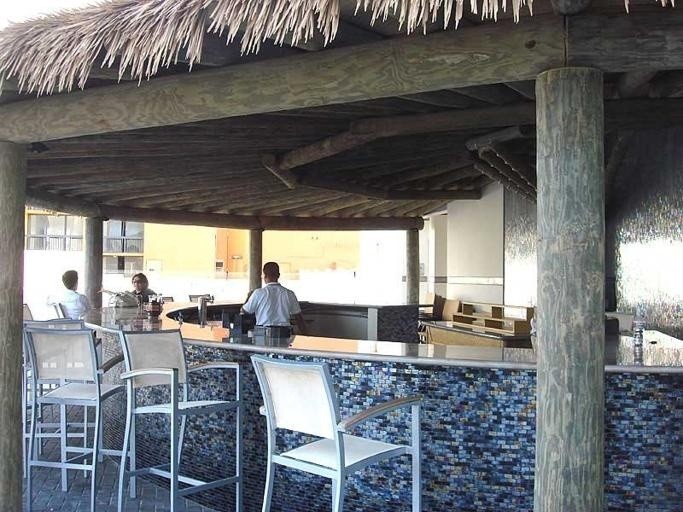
[452,302,534,336]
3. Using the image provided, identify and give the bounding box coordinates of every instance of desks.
[421,320,532,348]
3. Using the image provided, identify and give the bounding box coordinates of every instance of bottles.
[633,345,644,366]
[631,316,645,347]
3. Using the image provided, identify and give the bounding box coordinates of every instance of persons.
[129,272,156,304]
[45,270,93,323]
[239,262,314,337]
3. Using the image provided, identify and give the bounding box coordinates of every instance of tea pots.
[146,317,164,332]
[145,296,164,316]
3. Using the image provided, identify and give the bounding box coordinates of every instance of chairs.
[120,328,244,510]
[43,296,65,318]
[248,352,423,511]
[23,303,129,511]
